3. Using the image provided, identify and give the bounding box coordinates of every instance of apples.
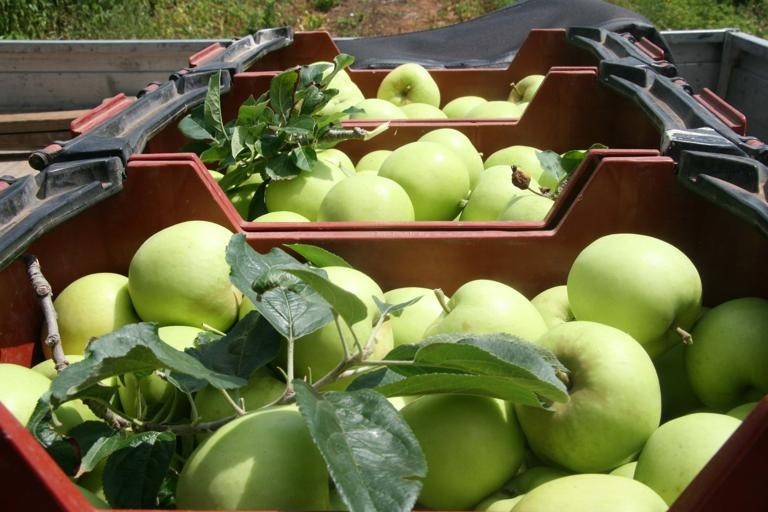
[297,63,546,121]
[0,220,768,511]
[207,128,589,221]
